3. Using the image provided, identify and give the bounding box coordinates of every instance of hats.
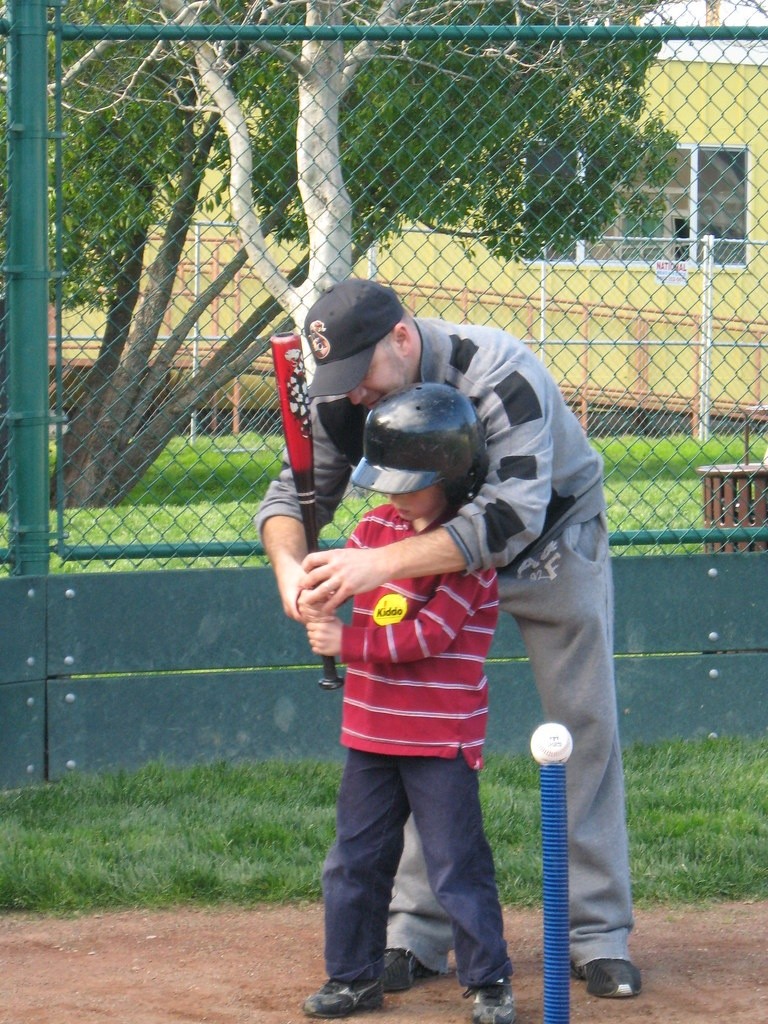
[304,279,403,398]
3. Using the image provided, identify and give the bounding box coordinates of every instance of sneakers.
[383,947,439,991]
[570,958,641,997]
[462,978,515,1024]
[301,975,384,1017]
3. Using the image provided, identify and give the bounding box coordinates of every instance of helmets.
[350,383,488,506]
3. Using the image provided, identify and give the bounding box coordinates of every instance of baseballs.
[529,722,573,766]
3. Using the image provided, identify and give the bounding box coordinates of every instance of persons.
[251,278,642,999]
[297,382,516,1024]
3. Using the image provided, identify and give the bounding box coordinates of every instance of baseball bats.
[270,333,345,692]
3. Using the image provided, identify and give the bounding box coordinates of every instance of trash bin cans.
[693,463,768,555]
